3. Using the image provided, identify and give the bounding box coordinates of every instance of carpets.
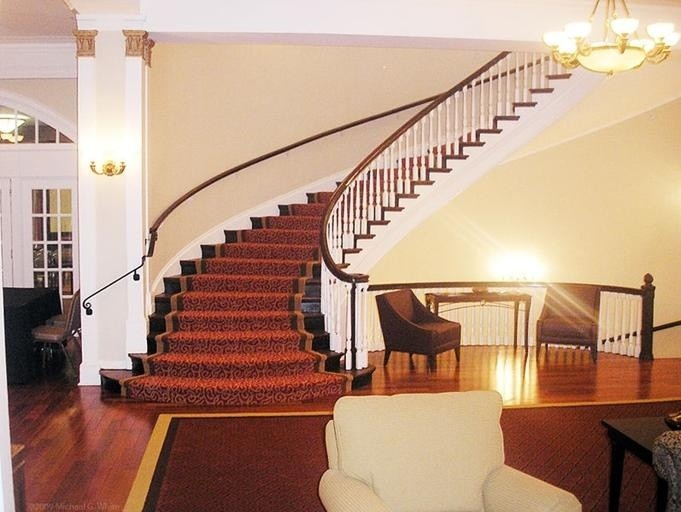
[122,396,680,512]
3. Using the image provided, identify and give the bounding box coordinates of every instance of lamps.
[542,0,681,76]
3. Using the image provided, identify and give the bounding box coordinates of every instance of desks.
[600,413,681,512]
[4,287,62,383]
[425,291,532,353]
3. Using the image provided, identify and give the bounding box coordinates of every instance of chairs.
[31,288,80,373]
[376,288,461,373]
[535,281,602,362]
[317,389,583,512]
